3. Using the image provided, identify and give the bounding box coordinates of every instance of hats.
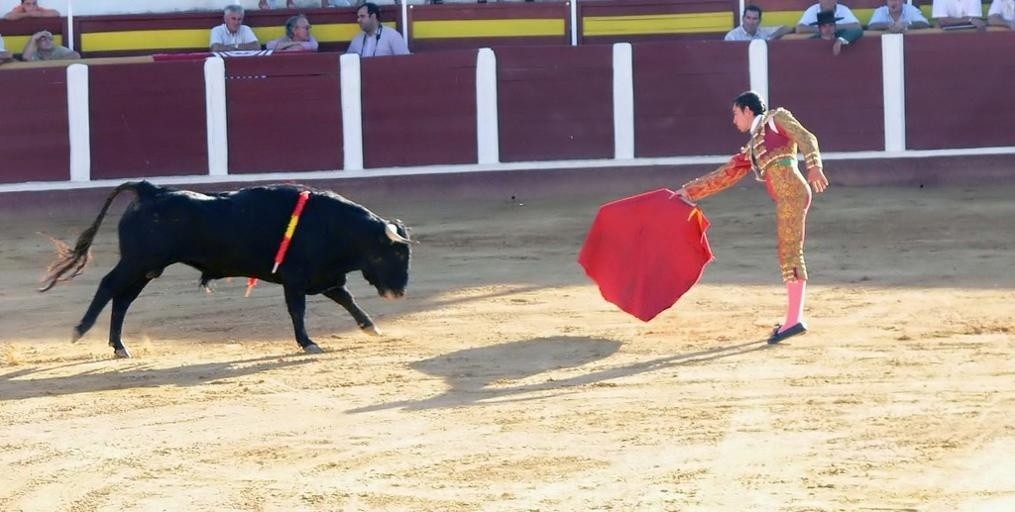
[809,9,845,26]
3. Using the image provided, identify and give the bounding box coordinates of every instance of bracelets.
[234,42,239,48]
[968,15,975,25]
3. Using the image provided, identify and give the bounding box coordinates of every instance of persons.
[932,0,986,32]
[258,1,536,11]
[266,15,318,51]
[20,29,81,61]
[805,10,863,56]
[723,5,795,41]
[345,3,409,58]
[795,0,862,34]
[208,4,262,51]
[987,0,1015,31]
[4,0,60,21]
[667,91,829,346]
[0,33,16,66]
[865,0,932,35]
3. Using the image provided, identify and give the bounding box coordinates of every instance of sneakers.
[767,321,807,345]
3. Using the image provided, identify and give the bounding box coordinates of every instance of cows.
[35,180,420,359]
